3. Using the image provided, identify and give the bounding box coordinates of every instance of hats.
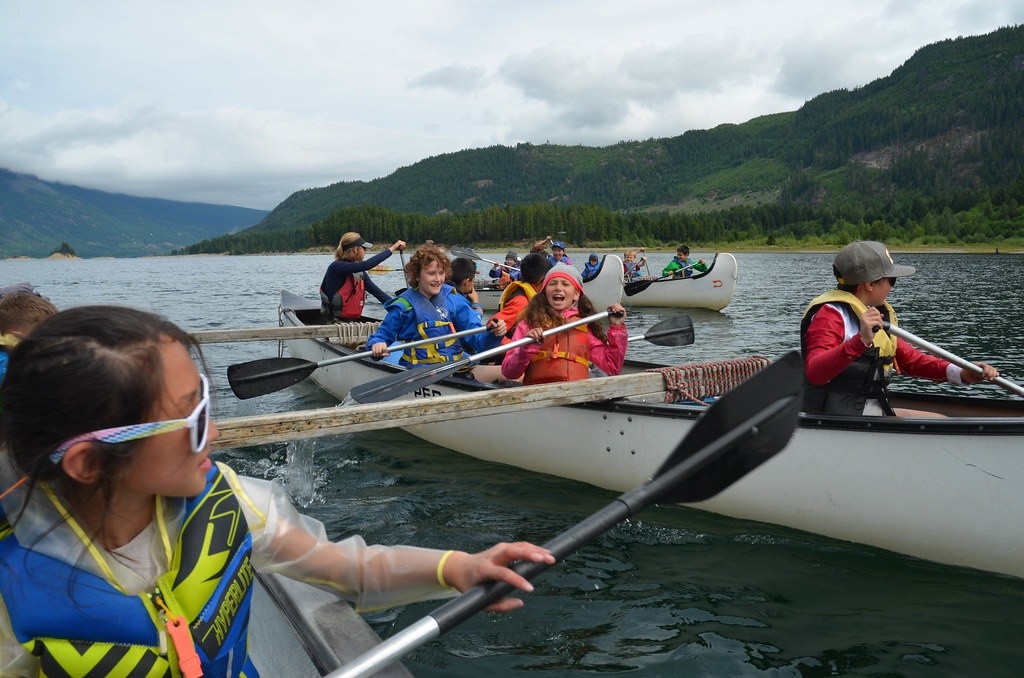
[341,237,373,250]
[551,241,565,250]
[832,240,916,285]
[541,262,584,295]
[505,251,517,261]
[589,254,598,261]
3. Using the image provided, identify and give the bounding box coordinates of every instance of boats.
[231,550,420,678]
[272,282,1024,583]
[616,252,740,312]
[473,254,626,313]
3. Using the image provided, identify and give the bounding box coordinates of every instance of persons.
[489,250,522,290]
[319,232,406,324]
[0,286,556,678]
[448,257,484,321]
[546,241,572,267]
[662,245,707,278]
[800,239,1000,418]
[622,250,647,278]
[366,244,525,384]
[581,254,601,279]
[488,253,553,355]
[501,263,629,386]
[530,236,552,258]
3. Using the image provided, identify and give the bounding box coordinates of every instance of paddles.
[349,308,625,405]
[394,244,412,290]
[366,264,408,275]
[628,314,696,347]
[873,318,1023,397]
[642,251,650,275]
[317,350,809,678]
[623,260,701,297]
[623,257,643,277]
[451,245,522,272]
[226,315,499,401]
[548,237,554,245]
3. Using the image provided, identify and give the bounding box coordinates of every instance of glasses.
[884,276,897,286]
[49,374,211,465]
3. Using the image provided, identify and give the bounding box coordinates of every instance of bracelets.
[437,550,455,592]
